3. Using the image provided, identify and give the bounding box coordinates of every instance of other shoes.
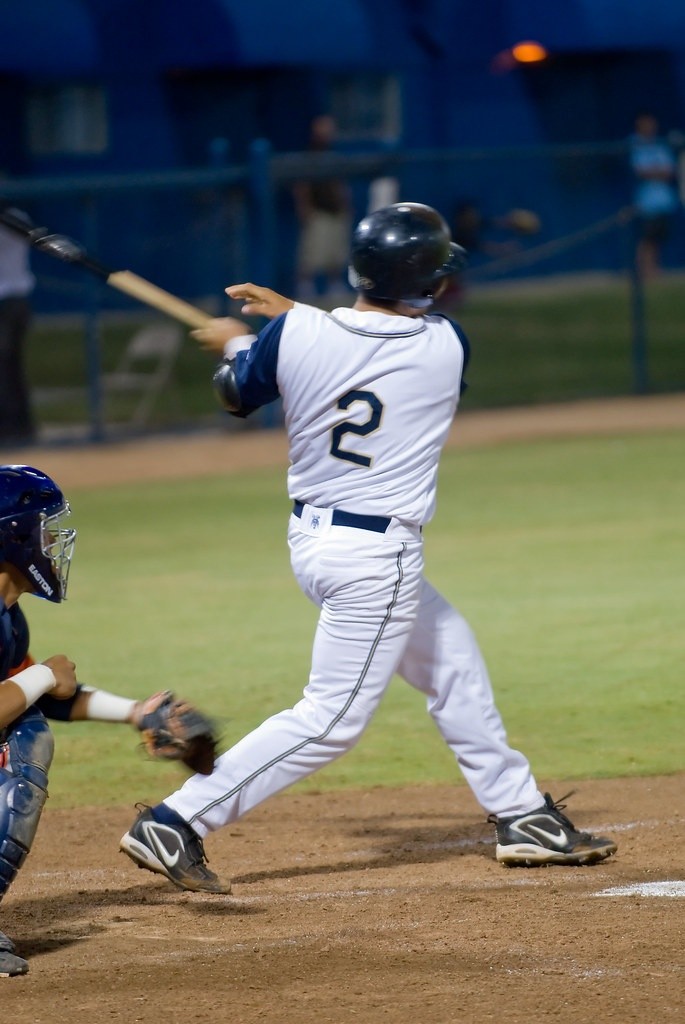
[0,951,29,978]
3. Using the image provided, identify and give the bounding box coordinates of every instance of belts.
[291,499,422,534]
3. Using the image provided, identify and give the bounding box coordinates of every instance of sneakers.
[487,791,618,864]
[120,802,231,893]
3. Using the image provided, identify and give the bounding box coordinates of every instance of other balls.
[513,43,547,61]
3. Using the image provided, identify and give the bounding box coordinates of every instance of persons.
[0,466,214,979]
[119,202,620,893]
[0,114,685,448]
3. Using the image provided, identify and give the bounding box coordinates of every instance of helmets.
[350,202,467,298]
[0,466,65,603]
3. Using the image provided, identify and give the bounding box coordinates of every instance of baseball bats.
[1,200,213,328]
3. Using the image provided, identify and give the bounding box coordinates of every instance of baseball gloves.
[136,691,217,777]
